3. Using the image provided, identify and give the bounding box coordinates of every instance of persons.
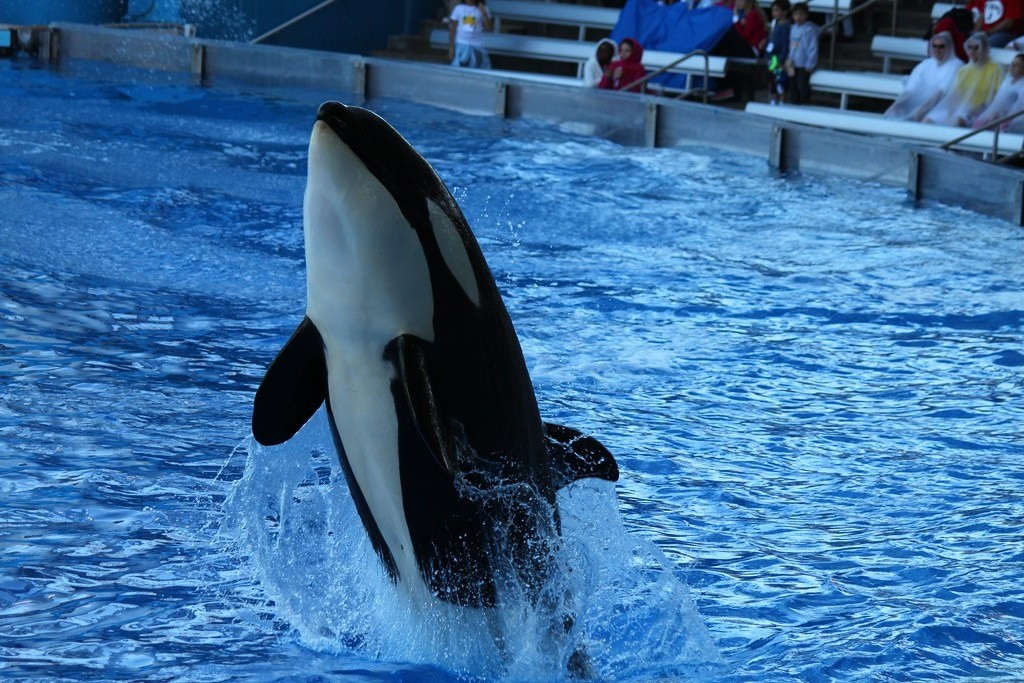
[708,0,768,101]
[924,30,1004,130]
[974,54,1024,134]
[583,37,621,91]
[884,31,965,124]
[925,0,1024,63]
[783,2,823,107]
[764,0,792,107]
[448,0,494,69]
[599,38,647,93]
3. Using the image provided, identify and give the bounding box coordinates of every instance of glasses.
[932,44,948,49]
[967,45,980,51]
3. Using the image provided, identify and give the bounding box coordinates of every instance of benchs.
[429,0,1023,110]
